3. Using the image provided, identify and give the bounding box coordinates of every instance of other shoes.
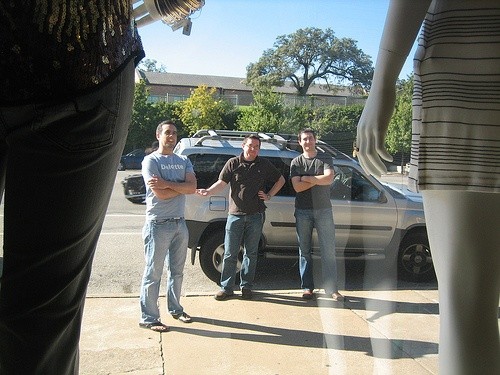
[173,312,192,323]
[241,287,253,299]
[214,290,235,300]
[138,322,168,332]
[332,292,345,302]
[302,289,314,299]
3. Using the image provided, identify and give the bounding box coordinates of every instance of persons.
[139,119,196,331]
[196,135,285,301]
[357,0,500,375]
[313,164,407,375]
[0,0,206,375]
[289,130,344,300]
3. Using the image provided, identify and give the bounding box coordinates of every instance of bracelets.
[267,194,271,198]
[153,0,205,27]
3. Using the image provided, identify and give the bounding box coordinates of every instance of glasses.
[245,143,259,151]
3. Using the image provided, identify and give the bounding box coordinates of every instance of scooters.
[121,174,148,204]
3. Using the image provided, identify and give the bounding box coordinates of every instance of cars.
[117,149,145,171]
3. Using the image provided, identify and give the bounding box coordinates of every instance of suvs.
[173,130,436,286]
